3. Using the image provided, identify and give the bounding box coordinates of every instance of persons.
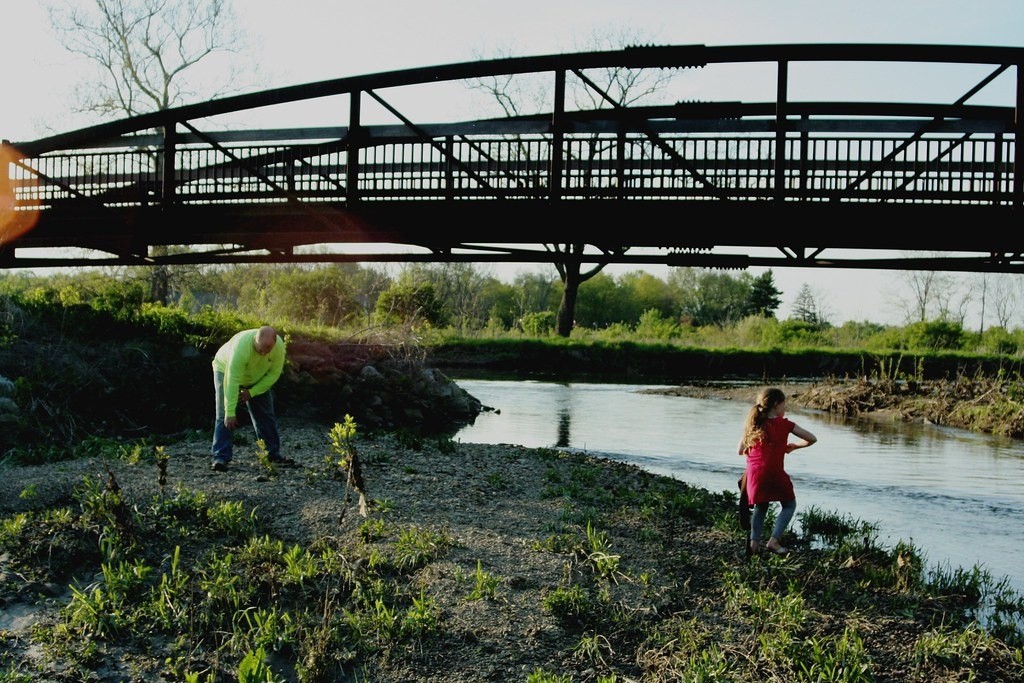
[212,326,296,474]
[739,386,817,557]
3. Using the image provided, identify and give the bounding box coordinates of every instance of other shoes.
[211,460,226,470]
[268,456,295,466]
[765,547,788,554]
